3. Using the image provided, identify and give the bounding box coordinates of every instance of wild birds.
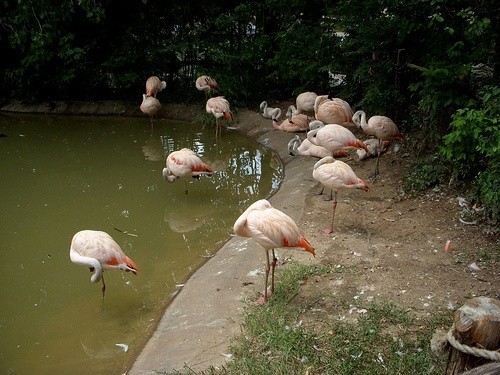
[260,101,274,119]
[287,138,348,201]
[140,94,162,124]
[285,91,317,124]
[144,76,167,98]
[314,95,361,126]
[271,108,316,133]
[307,120,374,159]
[196,75,221,107]
[312,156,370,236]
[356,138,391,161]
[68,229,141,298]
[162,148,217,194]
[206,95,233,138]
[233,199,316,301]
[352,110,404,185]
